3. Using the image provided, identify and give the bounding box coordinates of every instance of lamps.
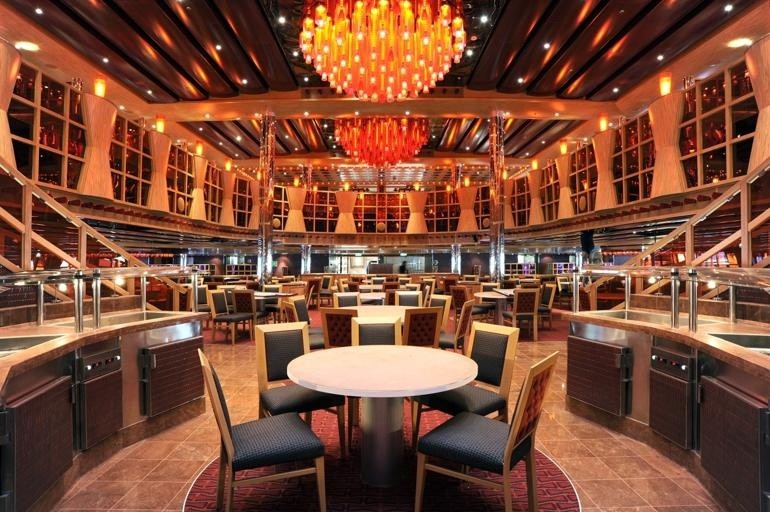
[300,0,467,169]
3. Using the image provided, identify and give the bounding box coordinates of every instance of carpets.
[185,397,580,510]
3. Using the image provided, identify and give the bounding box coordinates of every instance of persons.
[400,261,407,273]
